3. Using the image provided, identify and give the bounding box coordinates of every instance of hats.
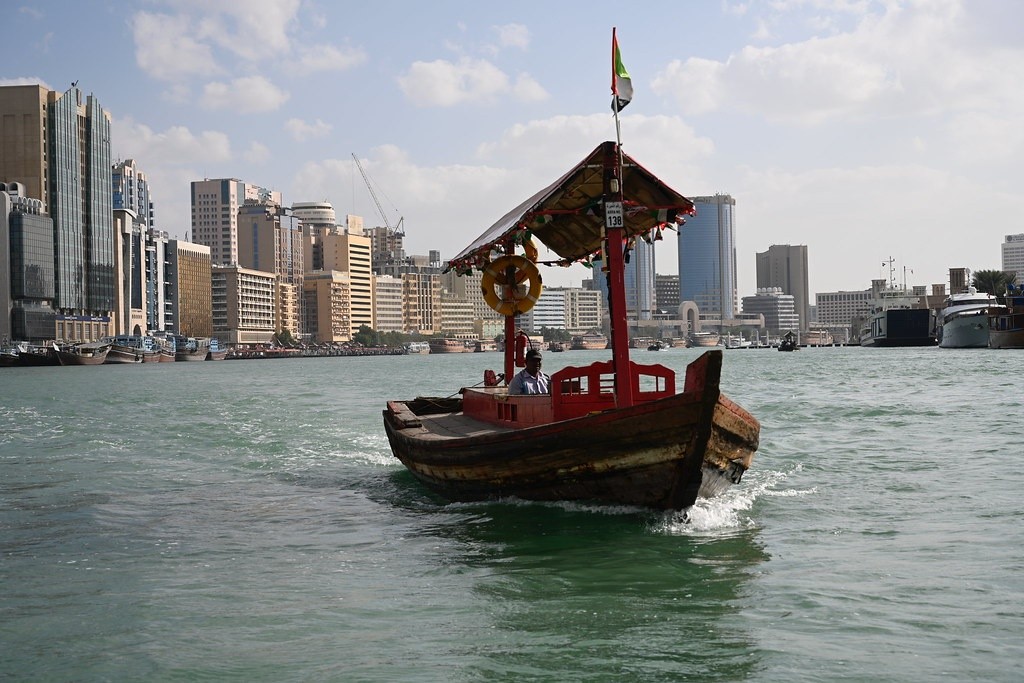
[526,349,542,359]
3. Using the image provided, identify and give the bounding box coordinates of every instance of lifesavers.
[480,255,543,317]
[480,238,538,286]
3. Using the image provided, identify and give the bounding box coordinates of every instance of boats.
[860,256,939,348]
[778,331,800,352]
[938,274,1024,349]
[647,340,670,352]
[430,331,832,353]
[687,345,694,348]
[0,333,228,366]
[402,341,431,355]
[552,347,568,353]
[380,141,760,527]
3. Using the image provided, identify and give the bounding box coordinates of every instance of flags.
[610,36,633,114]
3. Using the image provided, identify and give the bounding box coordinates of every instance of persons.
[509,350,549,394]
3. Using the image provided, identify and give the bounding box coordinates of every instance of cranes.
[352,151,405,254]
